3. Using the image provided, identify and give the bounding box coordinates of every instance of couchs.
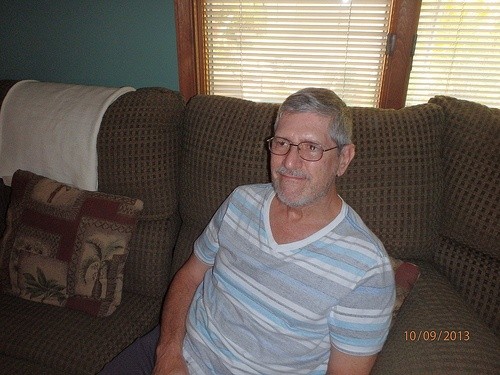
[0,77,499,375]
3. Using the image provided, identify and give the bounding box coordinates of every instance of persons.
[94,87,397,375]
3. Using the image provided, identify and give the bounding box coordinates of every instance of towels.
[0,79,137,192]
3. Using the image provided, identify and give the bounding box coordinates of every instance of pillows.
[1,168,144,318]
[388,254,420,325]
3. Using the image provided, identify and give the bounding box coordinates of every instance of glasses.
[267,136,351,162]
